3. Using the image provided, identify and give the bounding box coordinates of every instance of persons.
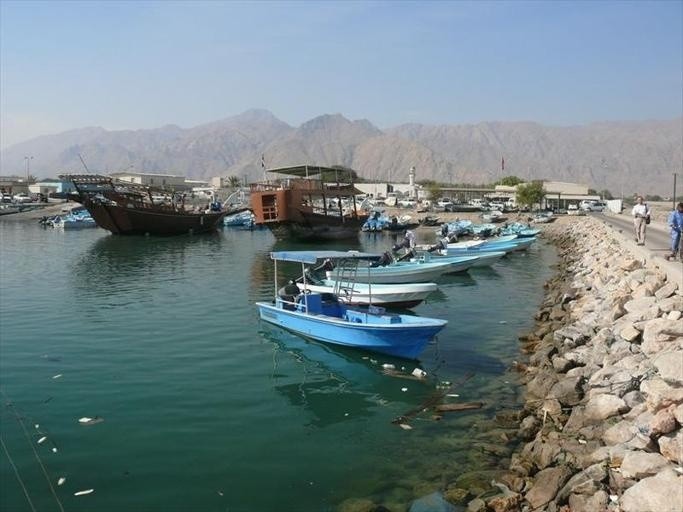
[632,196,652,246]
[669,203,683,256]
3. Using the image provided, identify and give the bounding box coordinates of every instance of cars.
[422,196,518,222]
[398,197,417,208]
[567,202,607,216]
[2,193,47,203]
[536,215,550,223]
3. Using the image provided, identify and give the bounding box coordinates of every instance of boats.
[325,257,477,280]
[291,276,437,311]
[38,210,96,232]
[249,164,372,238]
[416,212,534,267]
[49,175,241,237]
[256,244,447,362]
[362,213,438,233]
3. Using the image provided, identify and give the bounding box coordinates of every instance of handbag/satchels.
[644,204,650,224]
